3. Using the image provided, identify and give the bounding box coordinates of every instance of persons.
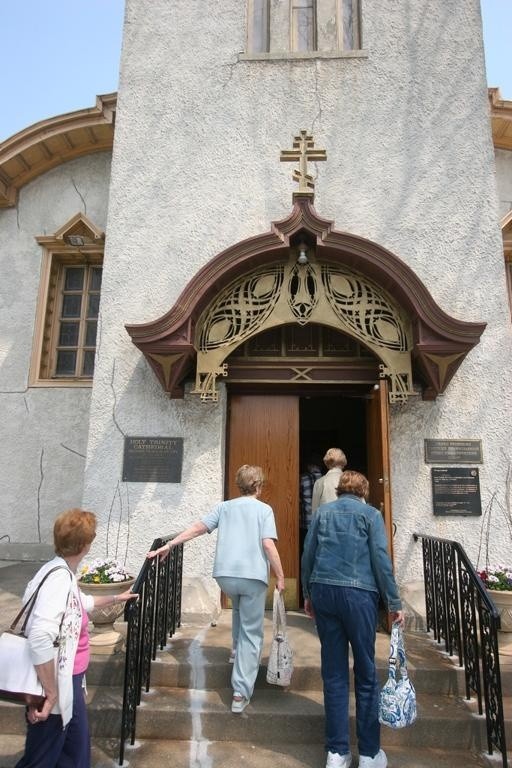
[301,470,405,768]
[302,459,322,529]
[16,508,140,767]
[313,447,347,513]
[146,465,284,713]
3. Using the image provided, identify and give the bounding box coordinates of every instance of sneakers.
[232,696,252,717]
[229,647,236,662]
[358,748,389,768]
[323,749,353,768]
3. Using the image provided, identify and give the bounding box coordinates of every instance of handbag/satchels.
[377,674,419,732]
[267,629,295,689]
[0,627,70,708]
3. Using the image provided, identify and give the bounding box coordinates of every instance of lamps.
[67,236,88,260]
[297,243,308,263]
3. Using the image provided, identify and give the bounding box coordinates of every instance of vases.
[78,575,136,646]
[476,588,512,654]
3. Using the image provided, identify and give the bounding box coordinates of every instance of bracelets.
[168,541,173,549]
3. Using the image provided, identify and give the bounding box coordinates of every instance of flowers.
[476,565,512,591]
[80,558,129,582]
